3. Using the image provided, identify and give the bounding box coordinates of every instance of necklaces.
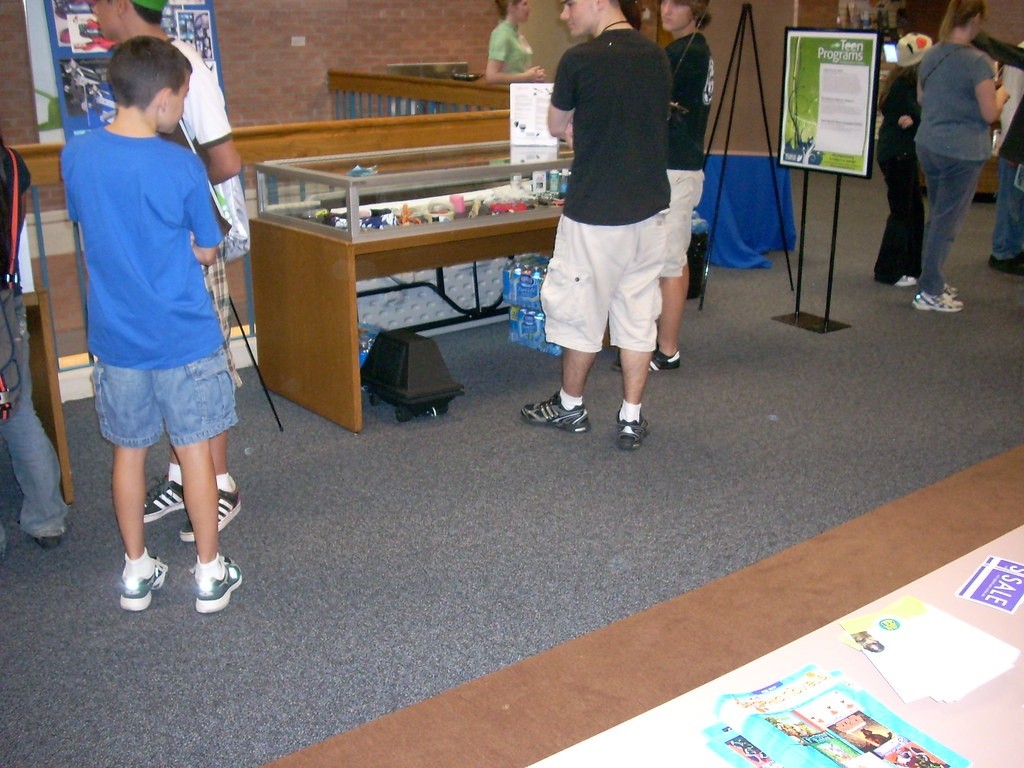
[603,21,629,32]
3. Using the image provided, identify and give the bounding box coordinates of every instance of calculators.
[452,72,483,82]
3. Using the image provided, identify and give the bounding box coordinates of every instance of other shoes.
[989,251,1024,276]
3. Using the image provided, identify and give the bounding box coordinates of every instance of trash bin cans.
[687,232,709,299]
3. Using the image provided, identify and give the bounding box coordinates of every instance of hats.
[896,33,933,66]
[132,0,168,11]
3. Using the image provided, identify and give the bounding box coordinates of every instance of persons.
[61,36,242,613]
[522,0,672,451]
[87,0,243,543]
[612,0,712,374]
[911,0,1010,313]
[486,0,546,84]
[874,31,932,287]
[0,132,68,562]
[971,30,1024,277]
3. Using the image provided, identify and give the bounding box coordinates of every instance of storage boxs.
[253,135,576,244]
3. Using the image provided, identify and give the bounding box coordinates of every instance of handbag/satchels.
[0,287,28,420]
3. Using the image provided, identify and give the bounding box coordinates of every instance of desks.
[246,216,559,437]
[699,148,797,272]
[17,278,74,505]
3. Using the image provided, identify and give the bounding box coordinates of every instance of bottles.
[501,252,563,357]
[357,322,385,367]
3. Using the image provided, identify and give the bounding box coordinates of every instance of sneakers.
[912,290,964,312]
[616,405,649,450]
[120,556,168,611]
[893,275,917,286]
[940,283,958,298]
[143,475,186,523]
[612,350,680,371]
[521,391,589,434]
[180,487,241,542]
[196,556,242,613]
